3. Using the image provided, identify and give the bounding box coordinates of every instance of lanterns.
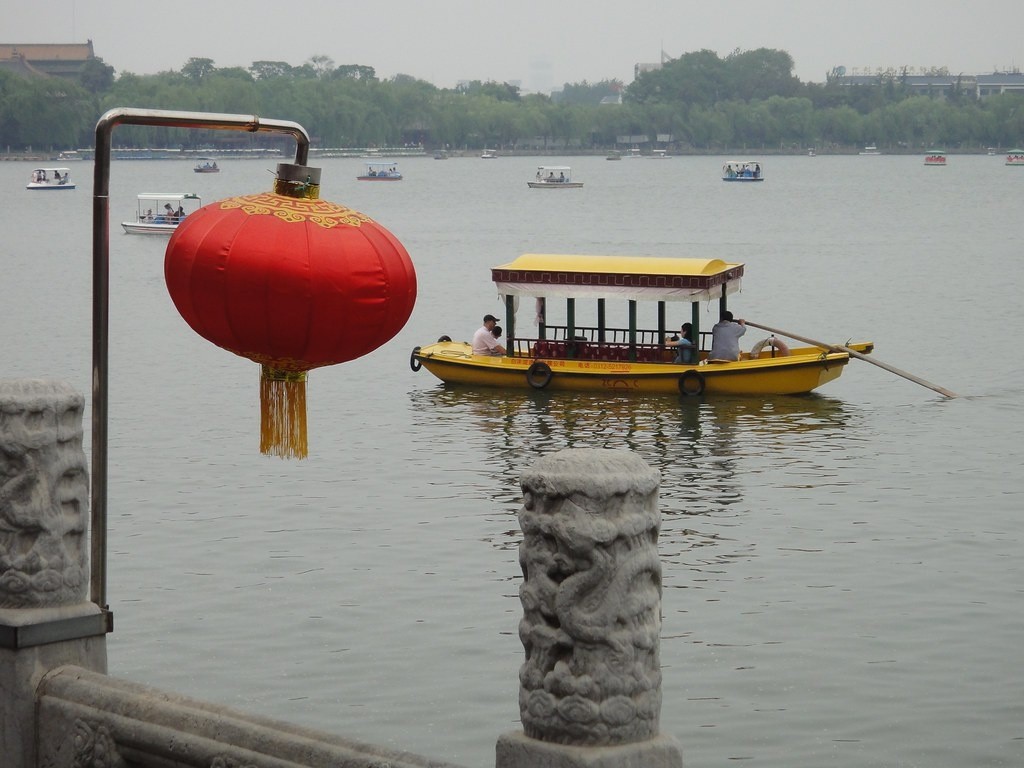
[162,180,417,458]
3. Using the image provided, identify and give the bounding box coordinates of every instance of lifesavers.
[750,337,790,360]
[437,335,452,343]
[410,346,422,372]
[526,362,553,389]
[678,369,705,397]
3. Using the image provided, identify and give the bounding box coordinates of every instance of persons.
[199,163,218,169]
[725,164,760,178]
[368,167,398,177]
[471,314,506,357]
[164,203,184,223]
[666,323,693,363]
[707,311,746,362]
[536,171,564,182]
[32,170,69,185]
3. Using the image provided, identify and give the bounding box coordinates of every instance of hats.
[484,315,500,322]
[493,326,502,336]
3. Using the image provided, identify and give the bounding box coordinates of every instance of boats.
[434,150,449,159]
[606,150,622,160]
[1005,148,1024,167]
[356,162,403,181]
[120,192,203,234]
[409,251,877,398]
[193,156,220,173]
[25,167,76,190]
[924,150,946,166]
[721,160,764,181]
[526,165,584,188]
[623,148,642,158]
[646,149,673,159]
[481,149,498,158]
[56,150,83,161]
